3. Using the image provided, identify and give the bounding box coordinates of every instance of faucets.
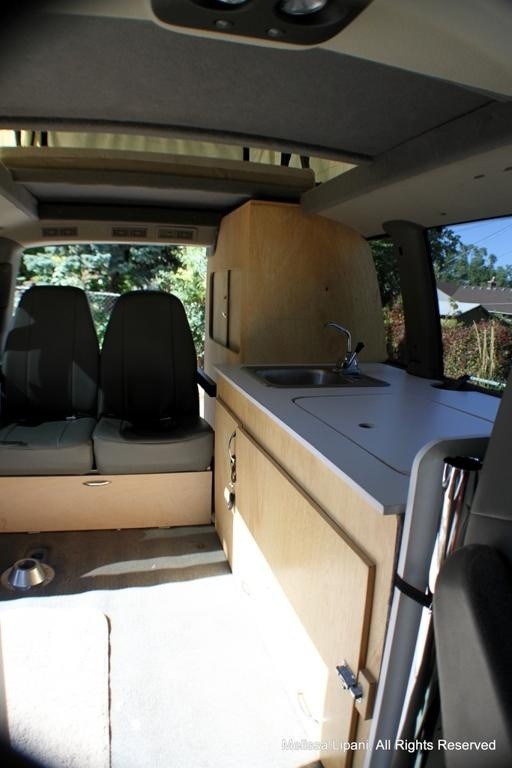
[323,321,365,374]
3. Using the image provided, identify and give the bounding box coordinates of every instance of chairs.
[1,276,103,478]
[91,284,216,475]
[369,371,511,768]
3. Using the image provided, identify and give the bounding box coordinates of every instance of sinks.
[241,365,391,388]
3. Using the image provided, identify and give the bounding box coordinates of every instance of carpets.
[0,523,323,767]
